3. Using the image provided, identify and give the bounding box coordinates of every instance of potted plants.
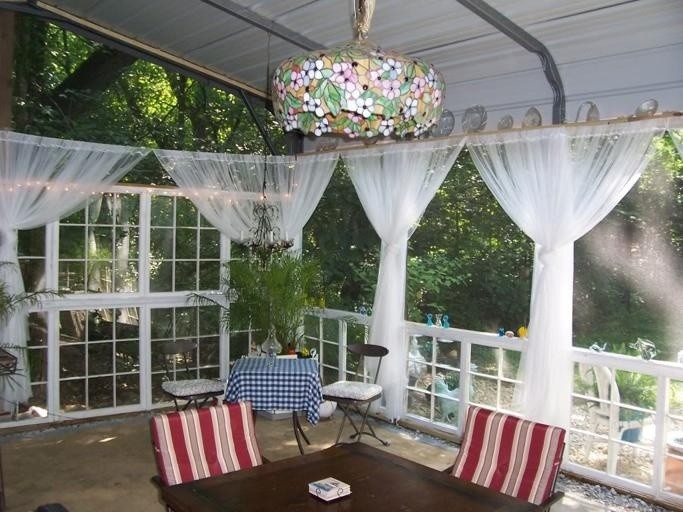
[186,246,324,421]
[589,337,680,443]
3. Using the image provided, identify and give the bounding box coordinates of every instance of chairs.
[159,339,225,412]
[578,349,656,478]
[148,400,270,511]
[321,343,390,446]
[442,405,566,511]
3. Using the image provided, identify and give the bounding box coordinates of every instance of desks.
[163,441,545,511]
[224,358,317,454]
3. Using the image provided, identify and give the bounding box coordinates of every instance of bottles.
[267,346,276,368]
[261,328,283,354]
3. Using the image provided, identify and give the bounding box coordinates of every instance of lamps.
[233,110,293,267]
[268,0,444,140]
